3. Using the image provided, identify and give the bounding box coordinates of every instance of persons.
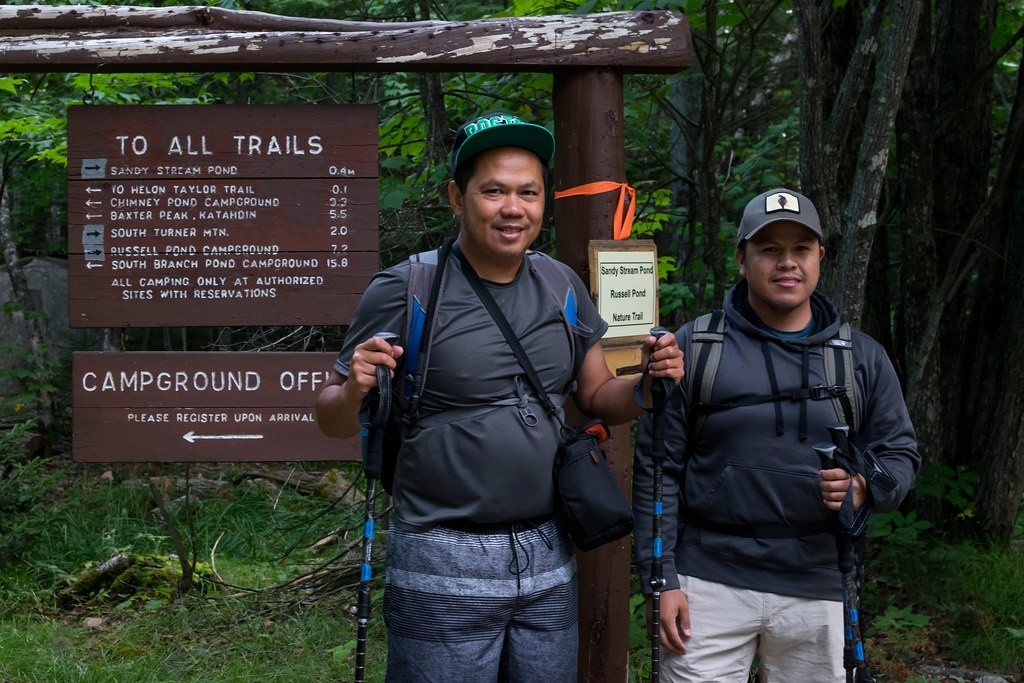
[318,109,684,683]
[631,188,922,683]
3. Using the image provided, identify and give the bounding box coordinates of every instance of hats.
[736,189,822,251]
[450,112,555,179]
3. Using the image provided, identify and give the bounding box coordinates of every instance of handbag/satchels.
[556,420,635,550]
